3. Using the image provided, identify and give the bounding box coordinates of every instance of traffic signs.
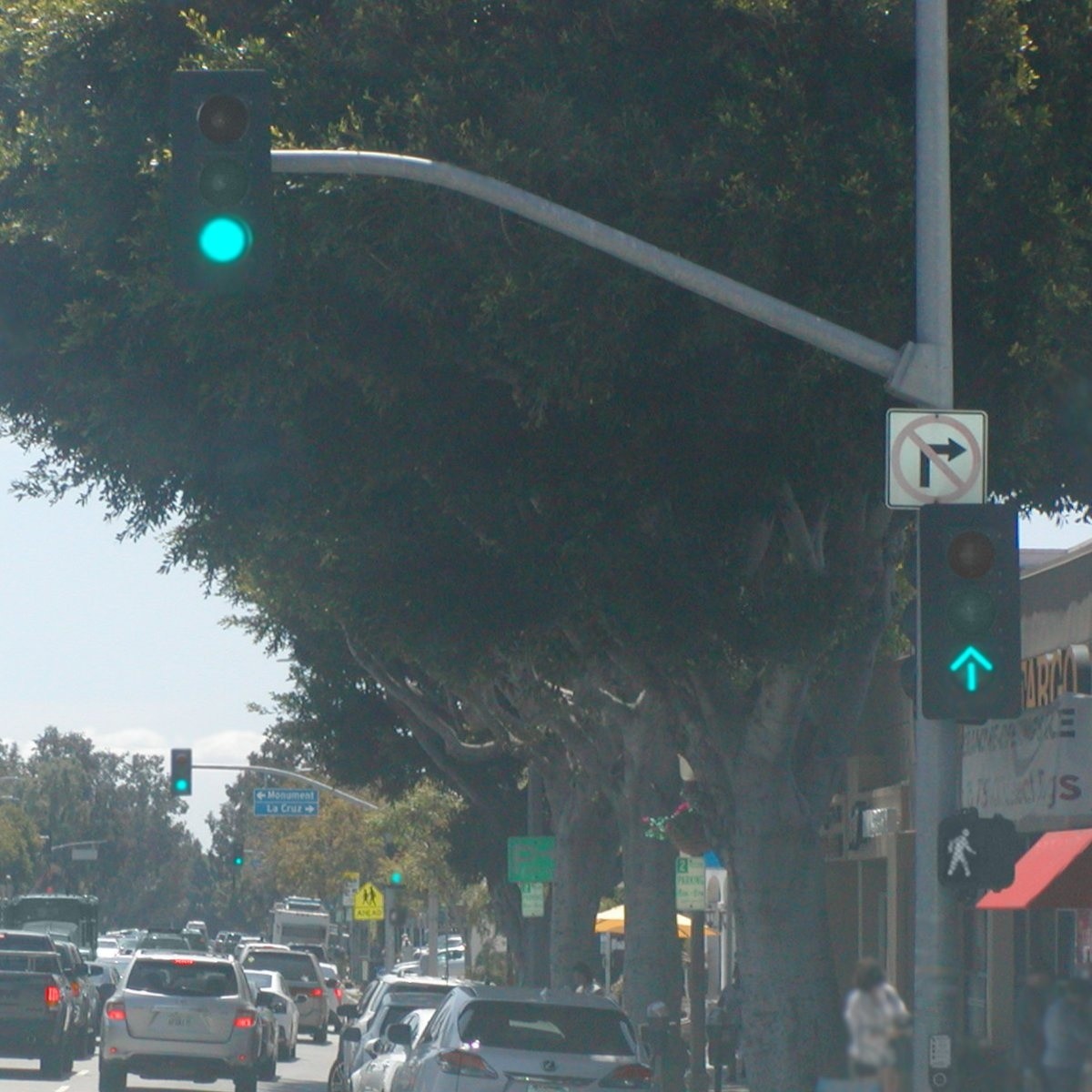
[253,803,318,816]
[253,789,320,803]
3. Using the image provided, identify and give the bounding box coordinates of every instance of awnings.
[975,829,1092,910]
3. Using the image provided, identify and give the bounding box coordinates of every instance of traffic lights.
[170,67,272,292]
[391,871,403,887]
[170,748,191,795]
[935,807,1016,893]
[918,499,1026,724]
[233,838,244,866]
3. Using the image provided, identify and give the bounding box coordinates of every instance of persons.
[646,1000,691,1092]
[718,965,747,1083]
[843,956,913,1092]
[370,933,440,977]
[327,941,348,980]
[571,961,604,996]
[1040,963,1092,1092]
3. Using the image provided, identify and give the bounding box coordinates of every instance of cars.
[386,984,656,1092]
[245,979,276,1082]
[244,970,308,1062]
[391,936,466,978]
[345,1009,438,1092]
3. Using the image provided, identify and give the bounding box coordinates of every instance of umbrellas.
[594,904,722,937]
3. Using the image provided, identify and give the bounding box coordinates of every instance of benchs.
[146,971,233,996]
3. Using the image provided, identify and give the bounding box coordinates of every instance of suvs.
[99,949,276,1092]
[240,945,338,1043]
[0,891,364,1034]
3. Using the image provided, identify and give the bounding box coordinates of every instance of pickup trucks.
[0,928,89,1080]
